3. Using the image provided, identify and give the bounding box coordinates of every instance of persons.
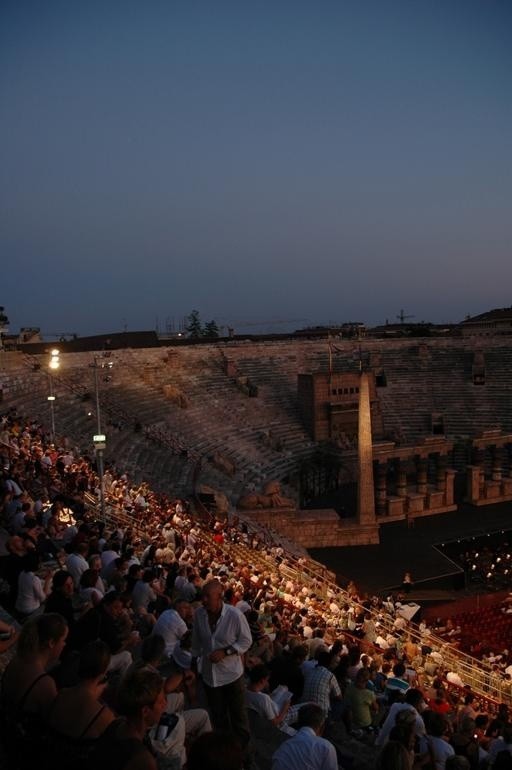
[2,412,511,770]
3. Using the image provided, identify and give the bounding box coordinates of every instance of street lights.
[88,355,114,517]
[48,347,61,433]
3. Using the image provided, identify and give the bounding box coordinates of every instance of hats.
[171,650,192,669]
[267,601,274,606]
[164,523,171,528]
[174,590,193,602]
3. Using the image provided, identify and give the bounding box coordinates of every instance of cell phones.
[0,632,11,638]
